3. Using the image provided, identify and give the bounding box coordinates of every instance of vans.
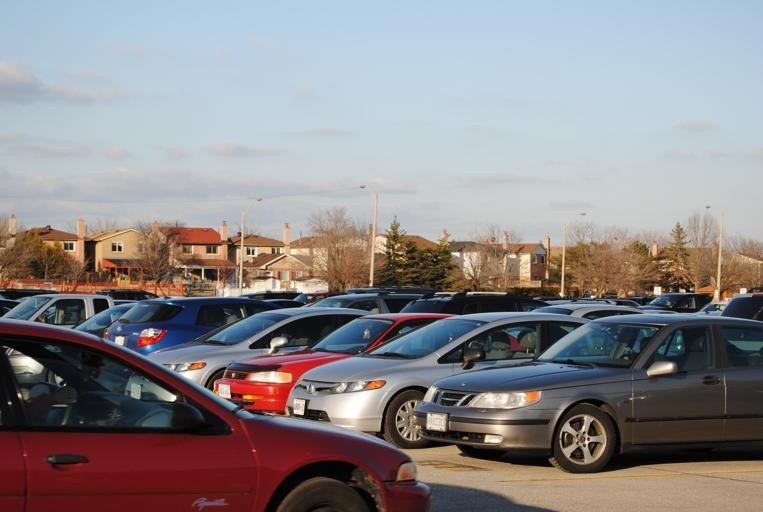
[0,294,113,330]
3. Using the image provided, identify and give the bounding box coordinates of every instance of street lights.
[358,183,378,285]
[704,205,725,300]
[237,196,262,294]
[559,211,585,299]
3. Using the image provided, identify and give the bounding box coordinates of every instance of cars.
[411,315,762,473]
[144,307,376,394]
[70,304,133,337]
[284,311,591,447]
[0,290,160,316]
[211,313,455,416]
[246,290,763,355]
[0,318,430,511]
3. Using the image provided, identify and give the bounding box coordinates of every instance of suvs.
[102,299,281,357]
